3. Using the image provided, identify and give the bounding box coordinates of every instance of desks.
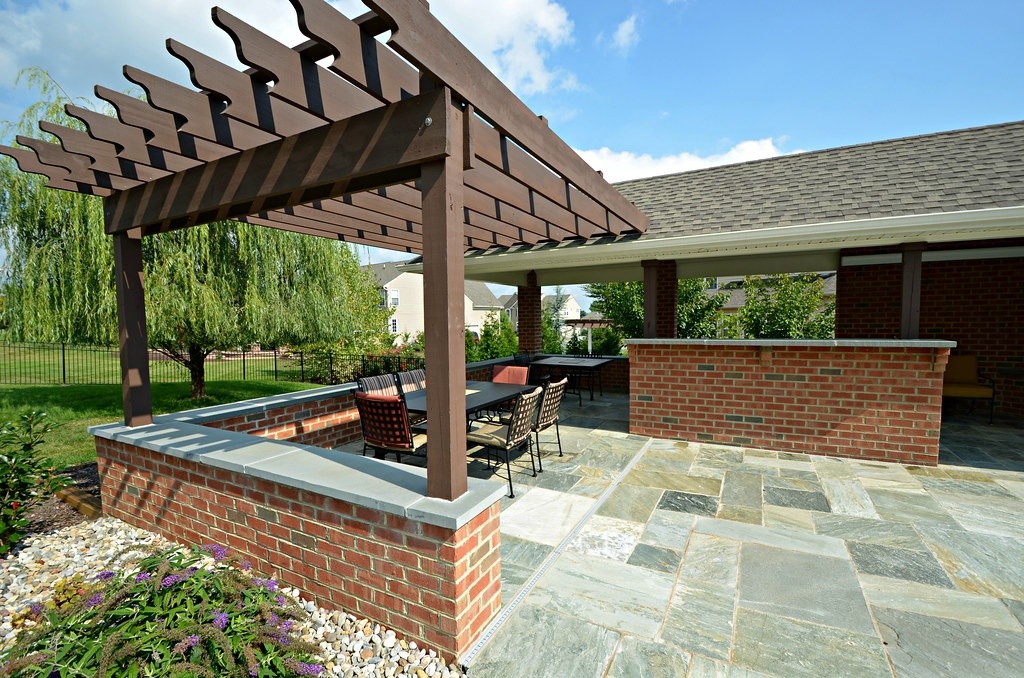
[531,357,613,407]
[393,380,537,436]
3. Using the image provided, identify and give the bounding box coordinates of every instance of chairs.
[476,365,531,417]
[513,351,549,380]
[943,353,996,425]
[357,369,426,423]
[352,391,427,464]
[466,377,567,498]
[566,349,604,396]
